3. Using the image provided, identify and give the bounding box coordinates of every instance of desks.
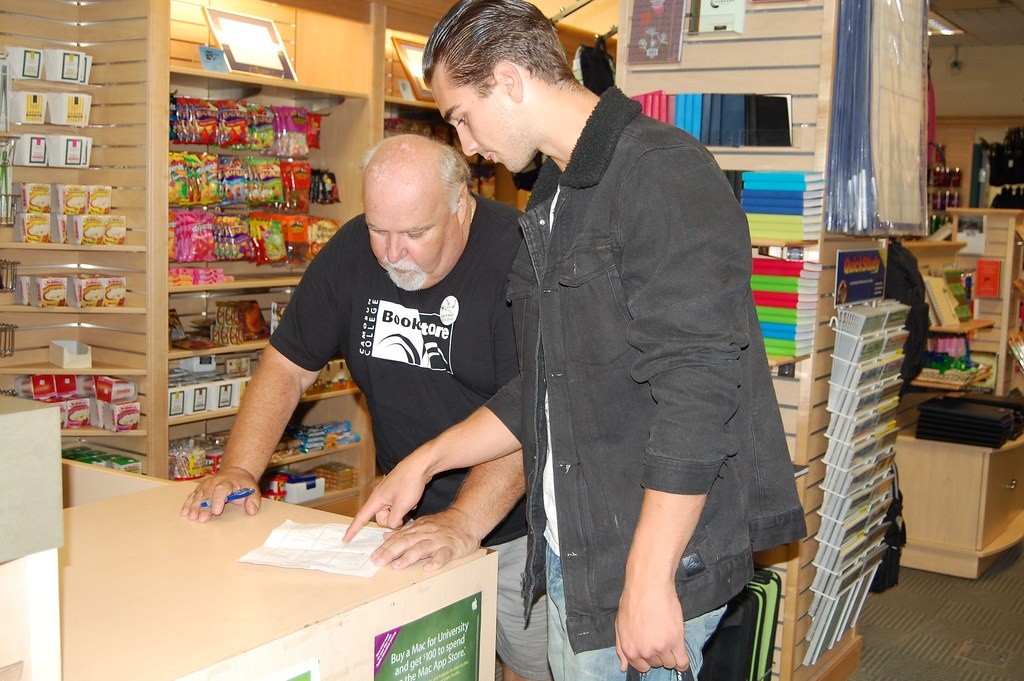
[58,455,498,681]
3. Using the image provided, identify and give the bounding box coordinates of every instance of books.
[976,259,1002,298]
[925,274,959,328]
[803,247,911,667]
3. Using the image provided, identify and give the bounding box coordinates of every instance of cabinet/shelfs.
[902,239,995,391]
[889,422,1024,579]
[0,0,612,516]
[947,208,1024,397]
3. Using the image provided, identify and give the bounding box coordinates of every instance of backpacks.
[884,239,930,379]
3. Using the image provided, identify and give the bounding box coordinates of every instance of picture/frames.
[202,6,299,81]
[391,36,436,103]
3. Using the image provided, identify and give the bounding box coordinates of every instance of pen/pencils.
[198,488,255,508]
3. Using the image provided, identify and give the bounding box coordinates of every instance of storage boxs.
[169,355,254,420]
[0,390,64,565]
[0,548,61,681]
[285,477,324,504]
[50,339,92,369]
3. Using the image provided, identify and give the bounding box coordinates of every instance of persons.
[182,135,553,681]
[886,236,927,402]
[342,1,807,681]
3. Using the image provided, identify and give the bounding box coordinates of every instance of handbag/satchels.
[868,462,906,593]
[987,127,1024,187]
